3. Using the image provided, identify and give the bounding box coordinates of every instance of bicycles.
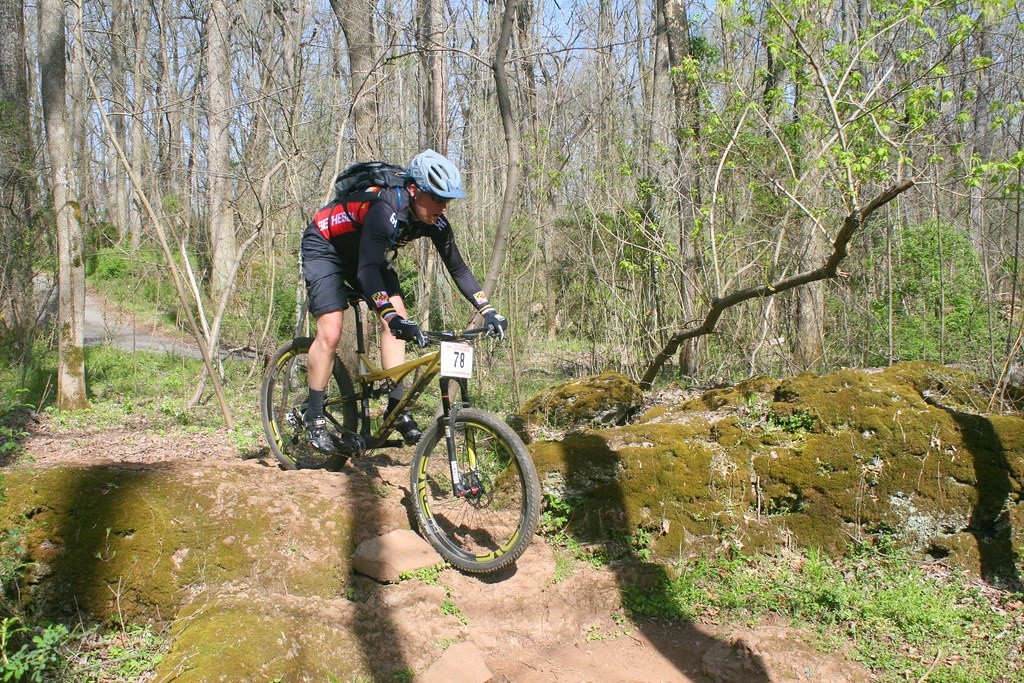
[260,323,542,573]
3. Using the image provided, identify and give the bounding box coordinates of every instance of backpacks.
[334,162,412,237]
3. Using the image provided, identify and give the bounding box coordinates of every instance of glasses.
[431,195,451,205]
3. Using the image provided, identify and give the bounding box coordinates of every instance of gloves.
[388,315,425,348]
[482,308,508,341]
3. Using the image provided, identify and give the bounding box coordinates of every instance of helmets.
[410,149,467,200]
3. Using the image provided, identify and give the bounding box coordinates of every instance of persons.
[299,148,508,454]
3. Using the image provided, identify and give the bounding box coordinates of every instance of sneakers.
[301,410,335,455]
[384,408,423,444]
